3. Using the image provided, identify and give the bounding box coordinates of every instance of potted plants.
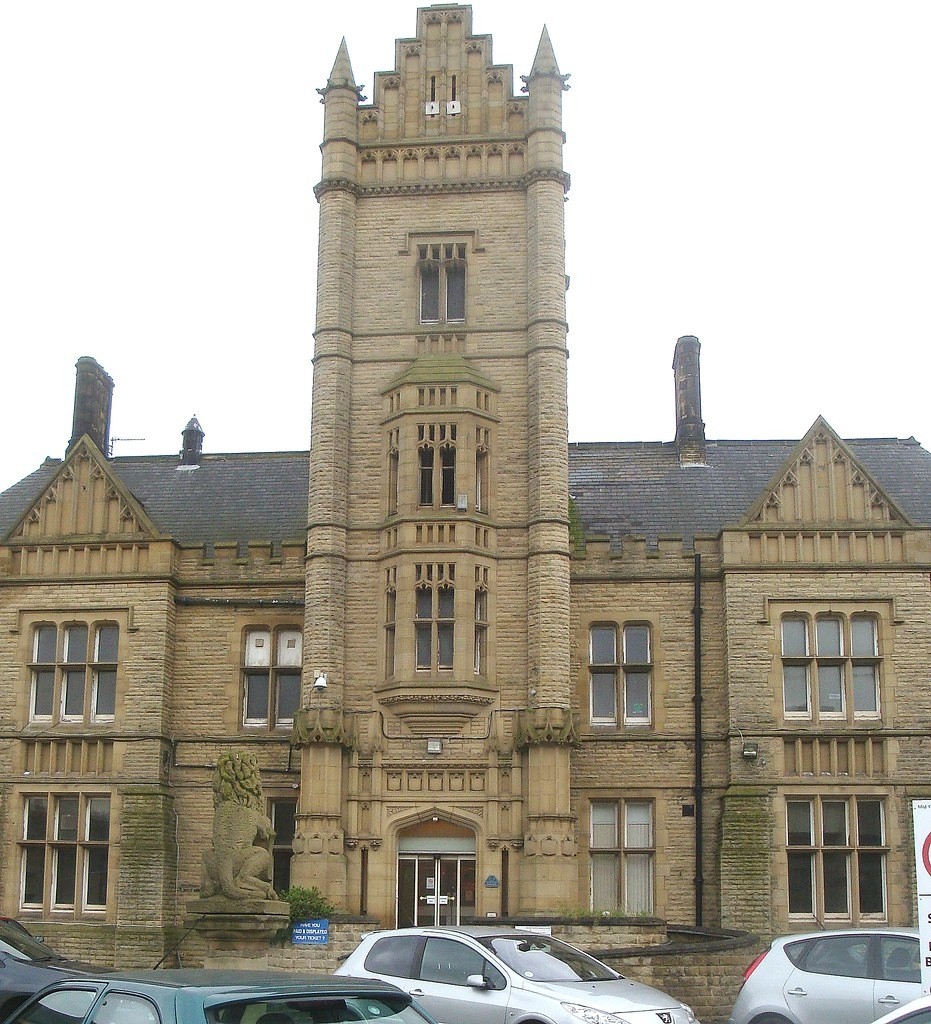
[278,884,334,921]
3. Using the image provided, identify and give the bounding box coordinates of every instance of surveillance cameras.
[315,676,328,691]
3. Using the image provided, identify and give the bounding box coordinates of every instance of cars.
[333,925,704,1024]
[0,914,117,1024]
[1,969,438,1024]
[727,927,921,1024]
[867,994,931,1024]
